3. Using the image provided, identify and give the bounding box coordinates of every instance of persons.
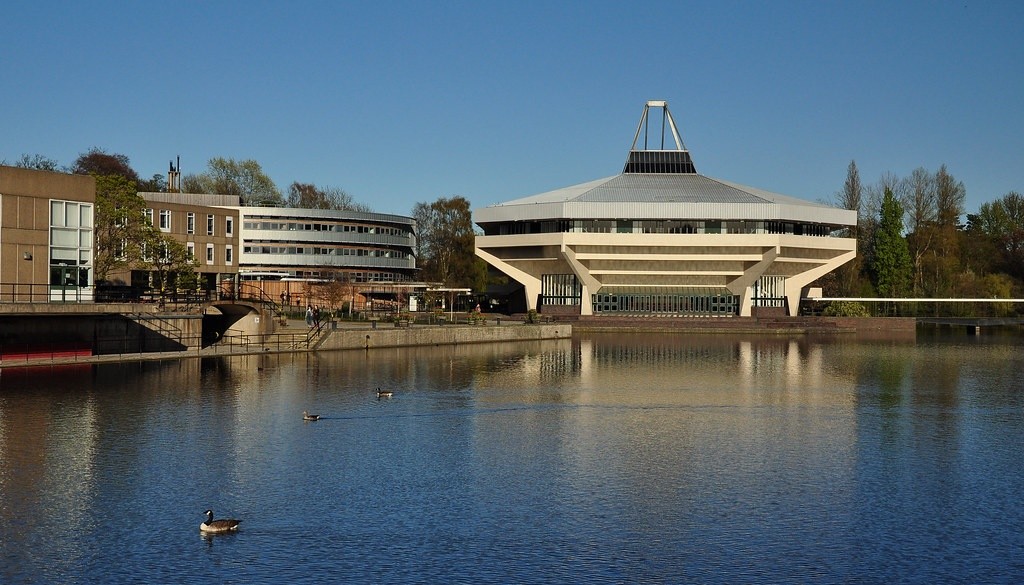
[306,305,319,326]
[280,291,285,304]
[475,304,480,312]
[296,296,302,310]
[286,293,291,305]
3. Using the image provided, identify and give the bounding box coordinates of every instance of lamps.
[24,252,31,259]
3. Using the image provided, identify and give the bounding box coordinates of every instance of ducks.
[375,387,393,397]
[199,510,242,531]
[257,366,263,371]
[302,411,320,420]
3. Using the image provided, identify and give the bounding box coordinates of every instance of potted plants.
[393,313,415,327]
[466,311,488,325]
